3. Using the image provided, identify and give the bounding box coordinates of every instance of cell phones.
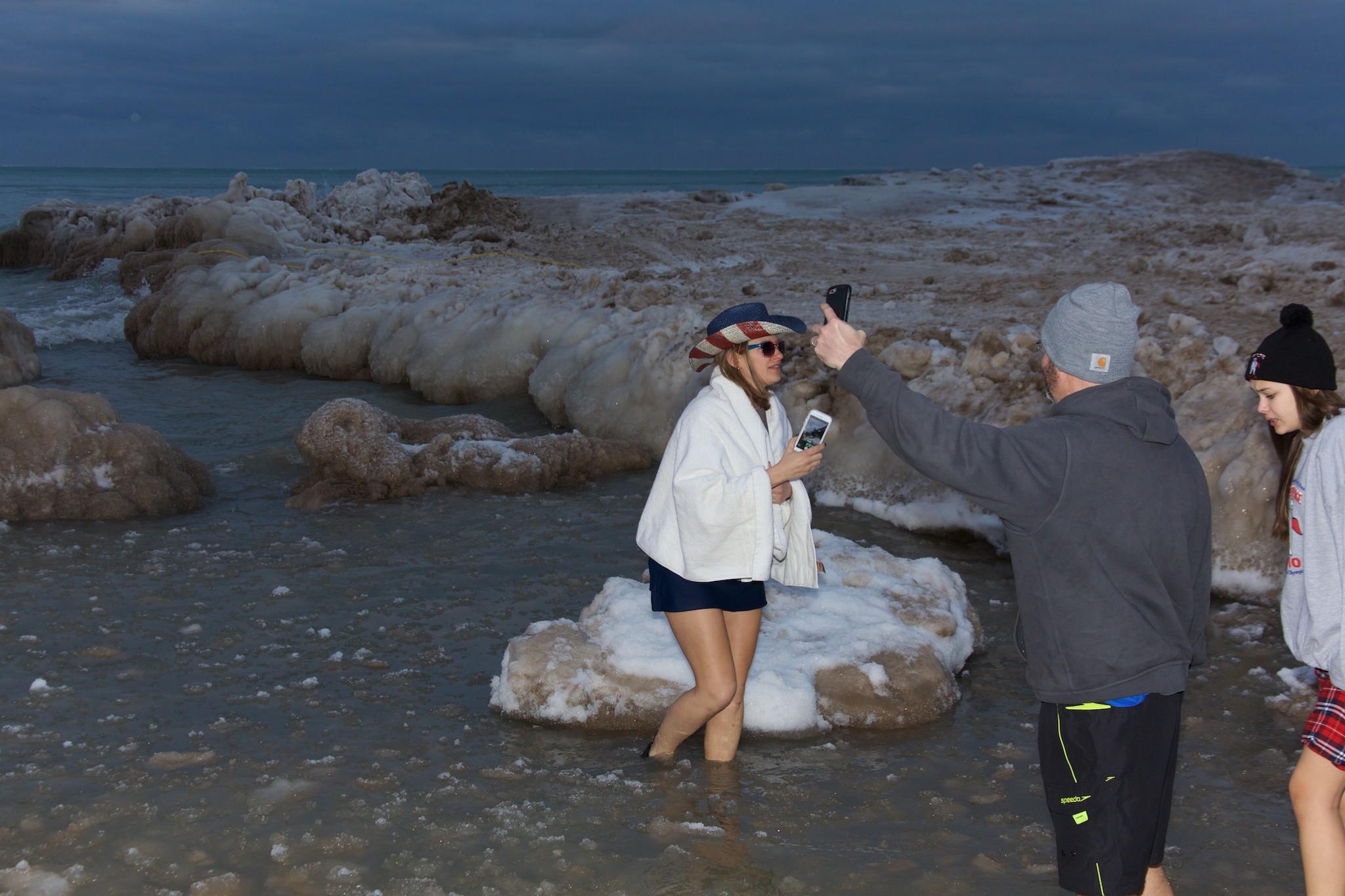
[823,285,851,325]
[794,408,831,453]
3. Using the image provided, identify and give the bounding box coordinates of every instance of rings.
[811,336,818,347]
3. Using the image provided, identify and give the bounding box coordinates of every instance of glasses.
[1036,339,1045,353]
[745,341,787,357]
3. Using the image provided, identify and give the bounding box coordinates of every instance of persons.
[810,282,1213,896]
[636,303,826,761]
[1246,303,1345,896]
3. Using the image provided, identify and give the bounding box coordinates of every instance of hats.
[1245,303,1338,390]
[688,302,806,373]
[1042,282,1138,383]
